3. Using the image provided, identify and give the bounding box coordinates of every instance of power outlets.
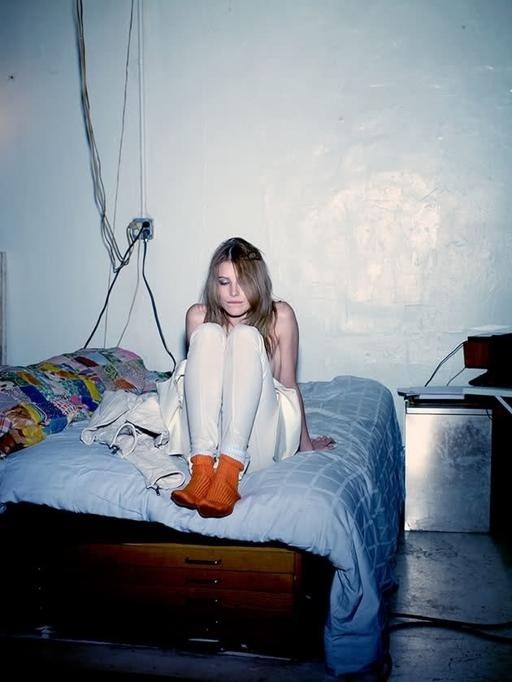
[130,217,155,242]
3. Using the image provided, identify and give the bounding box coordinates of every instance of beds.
[0,348,383,656]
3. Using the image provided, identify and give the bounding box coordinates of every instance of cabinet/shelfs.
[397,386,512,551]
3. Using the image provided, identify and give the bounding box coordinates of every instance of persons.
[170,237,336,518]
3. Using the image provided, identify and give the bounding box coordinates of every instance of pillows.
[0,348,145,460]
